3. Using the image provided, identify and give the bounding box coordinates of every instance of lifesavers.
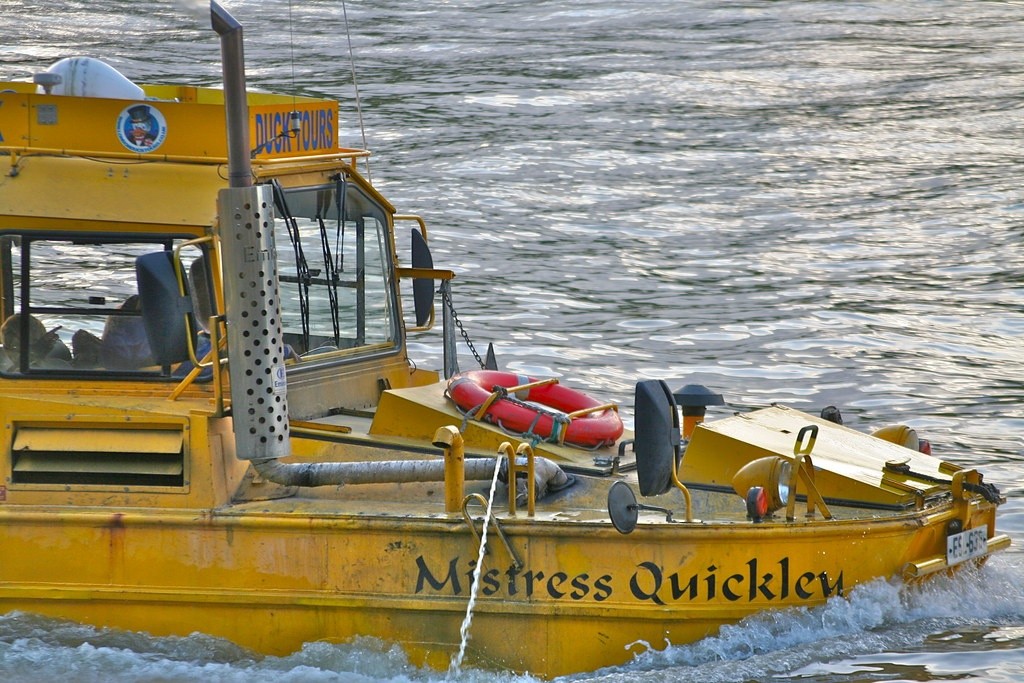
[448,368,624,447]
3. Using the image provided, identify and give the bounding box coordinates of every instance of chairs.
[1,338,70,372]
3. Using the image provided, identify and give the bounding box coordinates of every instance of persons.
[103,257,303,376]
[0,313,71,372]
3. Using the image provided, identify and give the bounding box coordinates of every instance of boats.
[1,0,1017,683]
